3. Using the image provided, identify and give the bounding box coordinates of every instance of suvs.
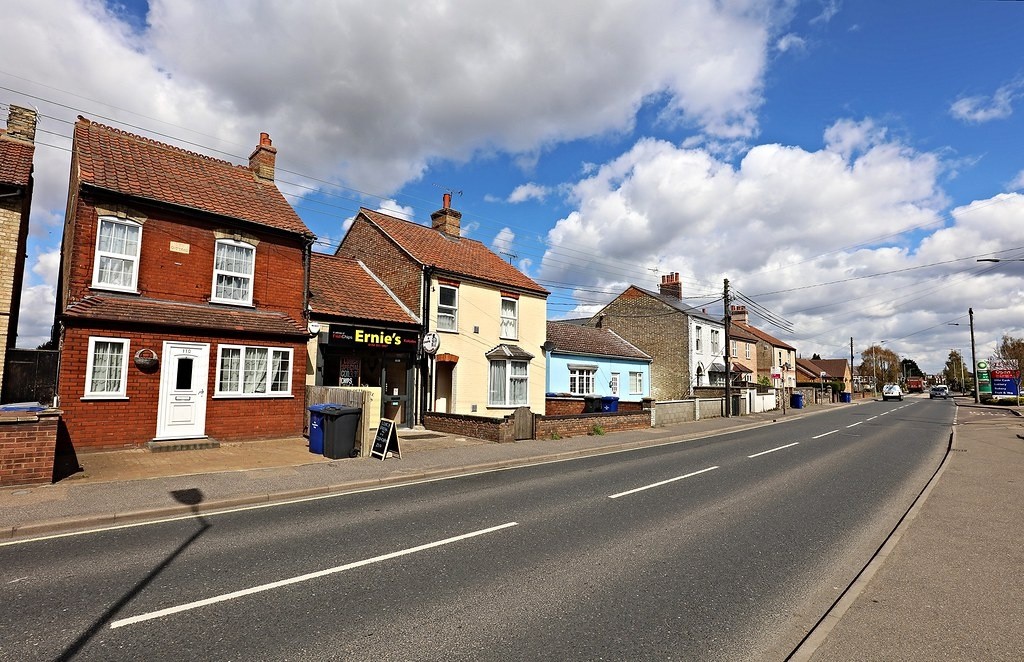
[882,385,904,401]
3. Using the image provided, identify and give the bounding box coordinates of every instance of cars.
[937,385,949,395]
[929,385,947,399]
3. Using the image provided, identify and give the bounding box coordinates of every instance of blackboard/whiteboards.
[371,418,401,456]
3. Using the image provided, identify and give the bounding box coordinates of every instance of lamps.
[609,381,613,388]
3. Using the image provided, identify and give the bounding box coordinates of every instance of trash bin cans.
[839,393,851,403]
[320,405,362,459]
[306,403,344,454]
[601,395,619,412]
[790,393,803,409]
[584,395,602,413]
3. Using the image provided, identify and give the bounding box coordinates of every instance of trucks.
[907,377,925,393]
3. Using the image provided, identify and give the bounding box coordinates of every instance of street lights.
[872,341,885,397]
[947,321,982,403]
